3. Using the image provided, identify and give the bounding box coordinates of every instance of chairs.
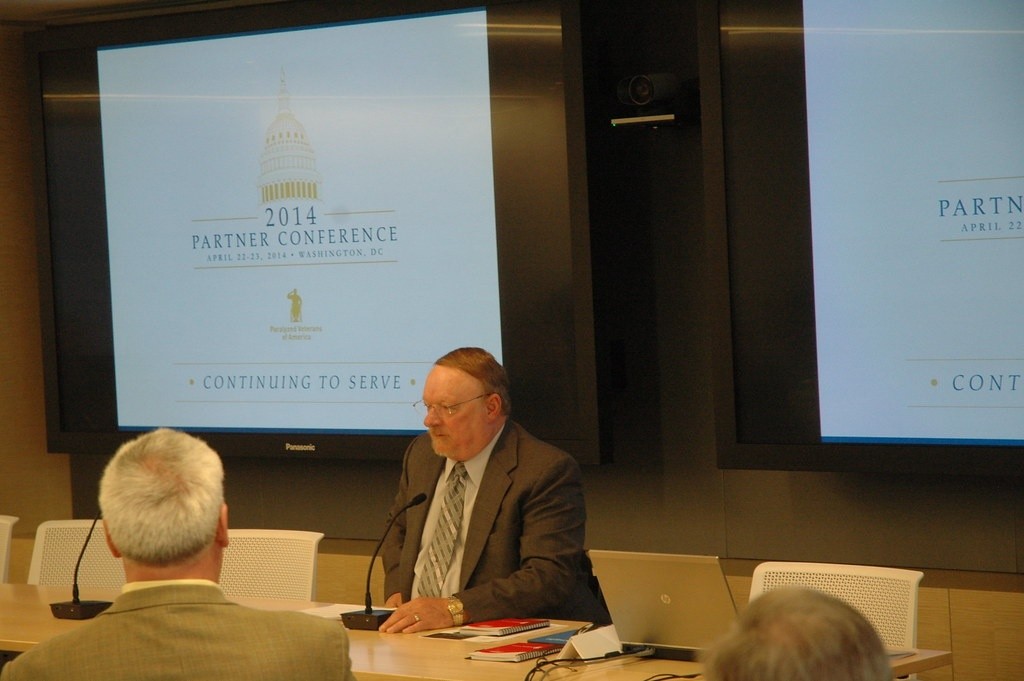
[28,519,127,586]
[217,527,324,601]
[747,562,925,680]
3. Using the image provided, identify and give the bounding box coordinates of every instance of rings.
[414,615,419,621]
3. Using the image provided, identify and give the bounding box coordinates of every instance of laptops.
[588,550,737,661]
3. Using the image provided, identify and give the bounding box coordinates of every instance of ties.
[418,462,467,597]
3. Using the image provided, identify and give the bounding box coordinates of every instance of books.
[468,642,565,662]
[459,618,550,637]
[528,630,578,643]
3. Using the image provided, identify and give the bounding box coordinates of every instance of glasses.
[413,393,503,417]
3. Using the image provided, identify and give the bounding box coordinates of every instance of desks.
[0,582,953,681]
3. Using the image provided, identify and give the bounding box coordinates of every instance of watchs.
[448,596,464,626]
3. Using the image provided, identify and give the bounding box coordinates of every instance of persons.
[710,585,892,681]
[0,430,356,681]
[378,347,611,634]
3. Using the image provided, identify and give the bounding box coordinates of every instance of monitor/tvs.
[691,0,1024,478]
[26,0,608,467]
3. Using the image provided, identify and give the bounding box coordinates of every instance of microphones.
[340,492,428,630]
